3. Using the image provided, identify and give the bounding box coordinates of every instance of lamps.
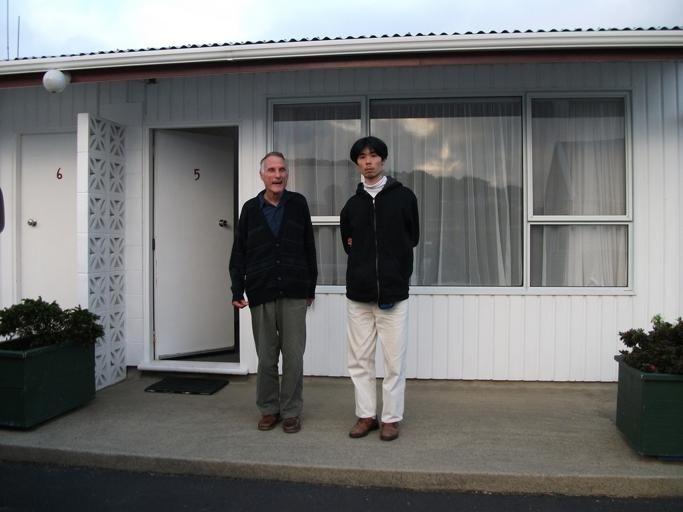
[43,70,72,93]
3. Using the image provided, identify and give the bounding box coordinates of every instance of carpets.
[143,374,229,394]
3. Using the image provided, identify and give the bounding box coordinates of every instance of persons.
[337,135,420,441]
[228,151,320,435]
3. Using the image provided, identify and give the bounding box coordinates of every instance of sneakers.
[380,422,400,440]
[258,411,282,430]
[350,414,380,437]
[281,415,301,432]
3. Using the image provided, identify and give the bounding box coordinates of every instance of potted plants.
[613,315,683,462]
[0,295,105,431]
